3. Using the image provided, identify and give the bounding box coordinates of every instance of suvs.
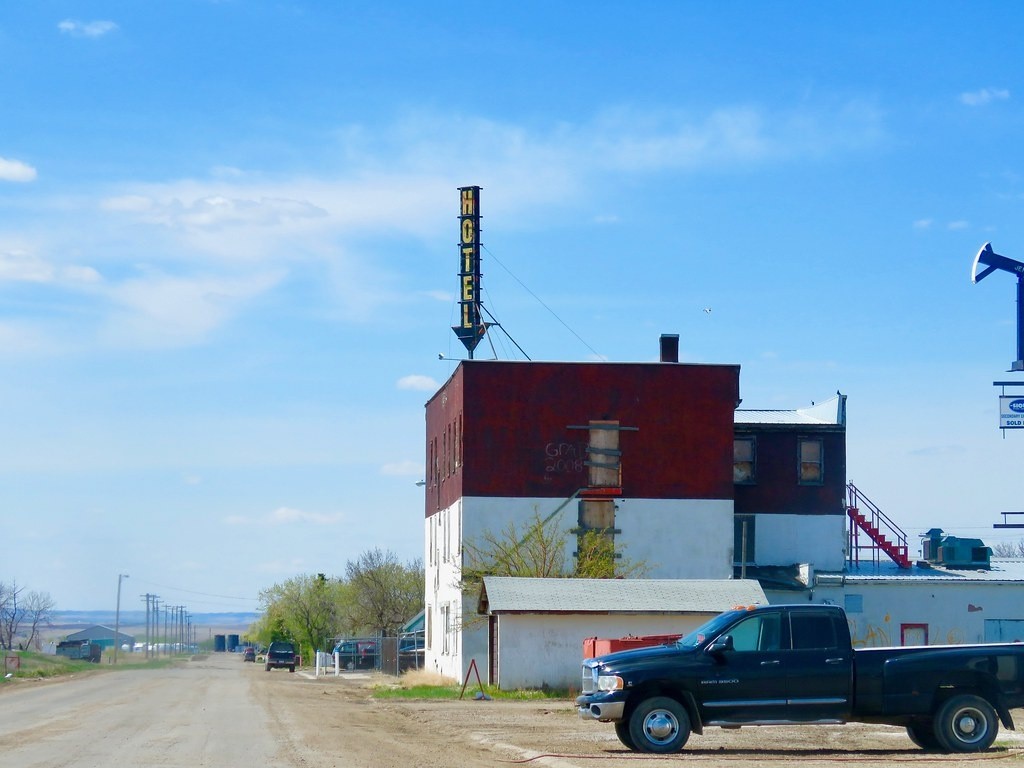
[244,648,254,653]
[332,636,375,670]
[264,642,296,673]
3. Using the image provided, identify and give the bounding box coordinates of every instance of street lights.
[114,574,129,664]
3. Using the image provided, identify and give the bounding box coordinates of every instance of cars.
[244,652,256,662]
[363,638,426,668]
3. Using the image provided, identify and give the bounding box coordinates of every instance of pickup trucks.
[573,602,1024,756]
